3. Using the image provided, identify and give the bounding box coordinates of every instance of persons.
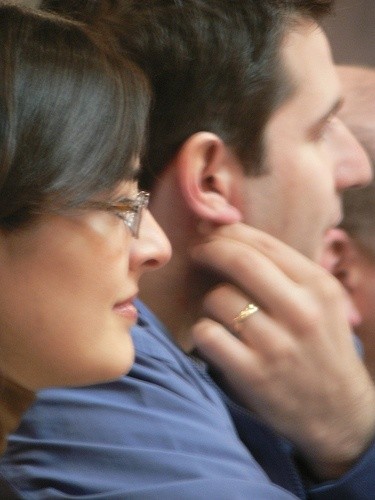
[0,0,374,499]
[0,0,175,500]
[325,64,375,385]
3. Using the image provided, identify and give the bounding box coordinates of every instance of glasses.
[40,191,151,236]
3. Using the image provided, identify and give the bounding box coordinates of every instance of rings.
[232,302,259,337]
